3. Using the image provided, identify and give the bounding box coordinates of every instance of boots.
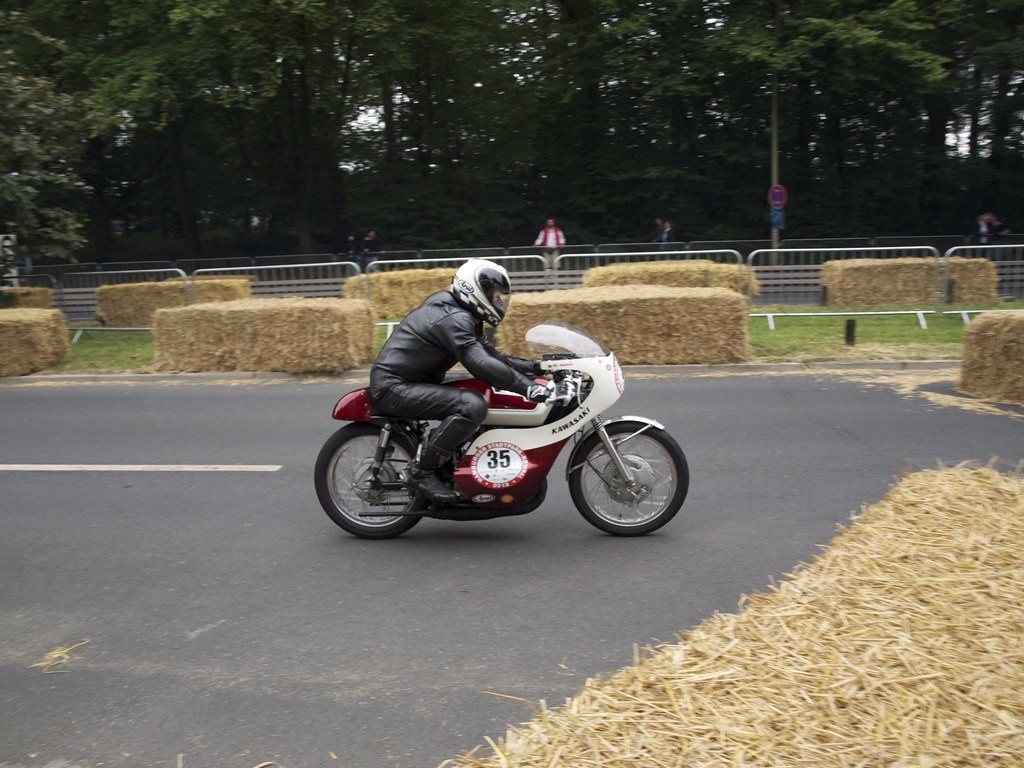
[399,439,457,504]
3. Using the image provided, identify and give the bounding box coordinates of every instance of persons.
[344,229,386,274]
[370,260,575,506]
[970,213,1009,257]
[531,218,565,280]
[10,240,44,286]
[648,218,675,261]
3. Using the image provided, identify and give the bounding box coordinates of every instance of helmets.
[450,258,511,328]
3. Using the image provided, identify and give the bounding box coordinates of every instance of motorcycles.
[310,317,692,539]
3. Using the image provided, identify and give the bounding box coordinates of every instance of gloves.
[527,383,554,403]
